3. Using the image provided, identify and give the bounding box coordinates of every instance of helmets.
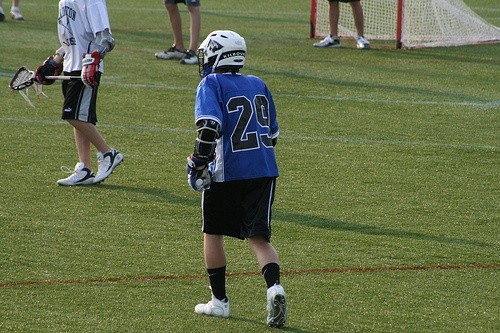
[196,30,247,81]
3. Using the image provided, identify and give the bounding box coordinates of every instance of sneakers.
[266,283,286,329]
[92,147,124,186]
[356,36,370,49]
[56,161,96,186]
[153,43,187,60]
[195,285,230,319]
[178,49,199,65]
[312,34,342,48]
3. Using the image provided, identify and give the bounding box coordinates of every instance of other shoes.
[0,6,4,15]
[10,8,24,20]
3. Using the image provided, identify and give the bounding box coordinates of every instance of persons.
[313,0,371,49]
[186,30,288,327]
[35,0,125,187]
[0,0,24,20]
[155,0,202,65]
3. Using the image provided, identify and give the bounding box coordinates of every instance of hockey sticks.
[10,66,81,90]
[197,177,206,186]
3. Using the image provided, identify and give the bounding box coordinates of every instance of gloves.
[34,55,64,86]
[185,153,212,192]
[80,51,102,88]
